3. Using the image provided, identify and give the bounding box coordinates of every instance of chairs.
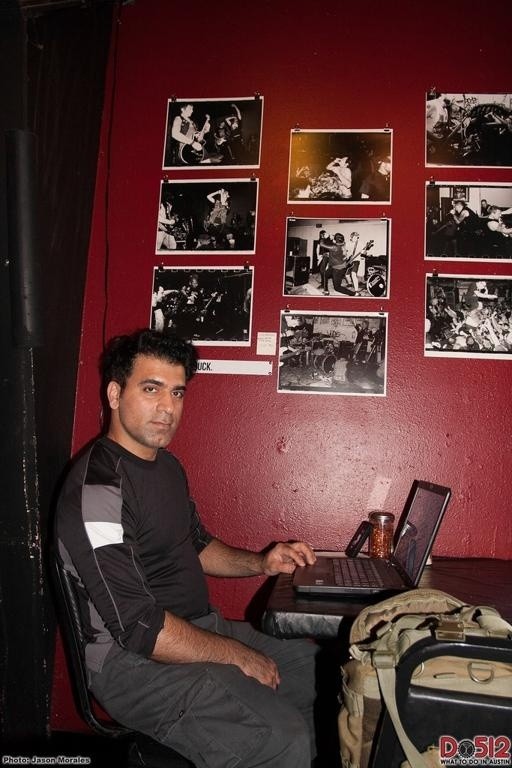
[367,635,512,767]
[47,538,194,768]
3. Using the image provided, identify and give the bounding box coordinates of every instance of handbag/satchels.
[337,587,511,768]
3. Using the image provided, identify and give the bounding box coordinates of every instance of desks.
[263,550,511,640]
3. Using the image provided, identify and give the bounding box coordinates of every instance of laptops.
[292,480,451,596]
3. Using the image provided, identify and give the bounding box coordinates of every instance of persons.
[169,100,242,164]
[155,187,255,250]
[54,329,316,767]
[289,129,392,202]
[425,181,512,259]
[280,313,386,394]
[427,274,512,356]
[151,269,252,341]
[286,217,389,299]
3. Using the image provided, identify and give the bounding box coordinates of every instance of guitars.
[346,240,374,274]
[427,111,467,162]
[179,114,212,165]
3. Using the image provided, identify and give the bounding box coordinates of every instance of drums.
[366,273,387,297]
[314,351,337,377]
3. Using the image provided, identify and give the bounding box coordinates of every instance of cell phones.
[345,521,373,558]
